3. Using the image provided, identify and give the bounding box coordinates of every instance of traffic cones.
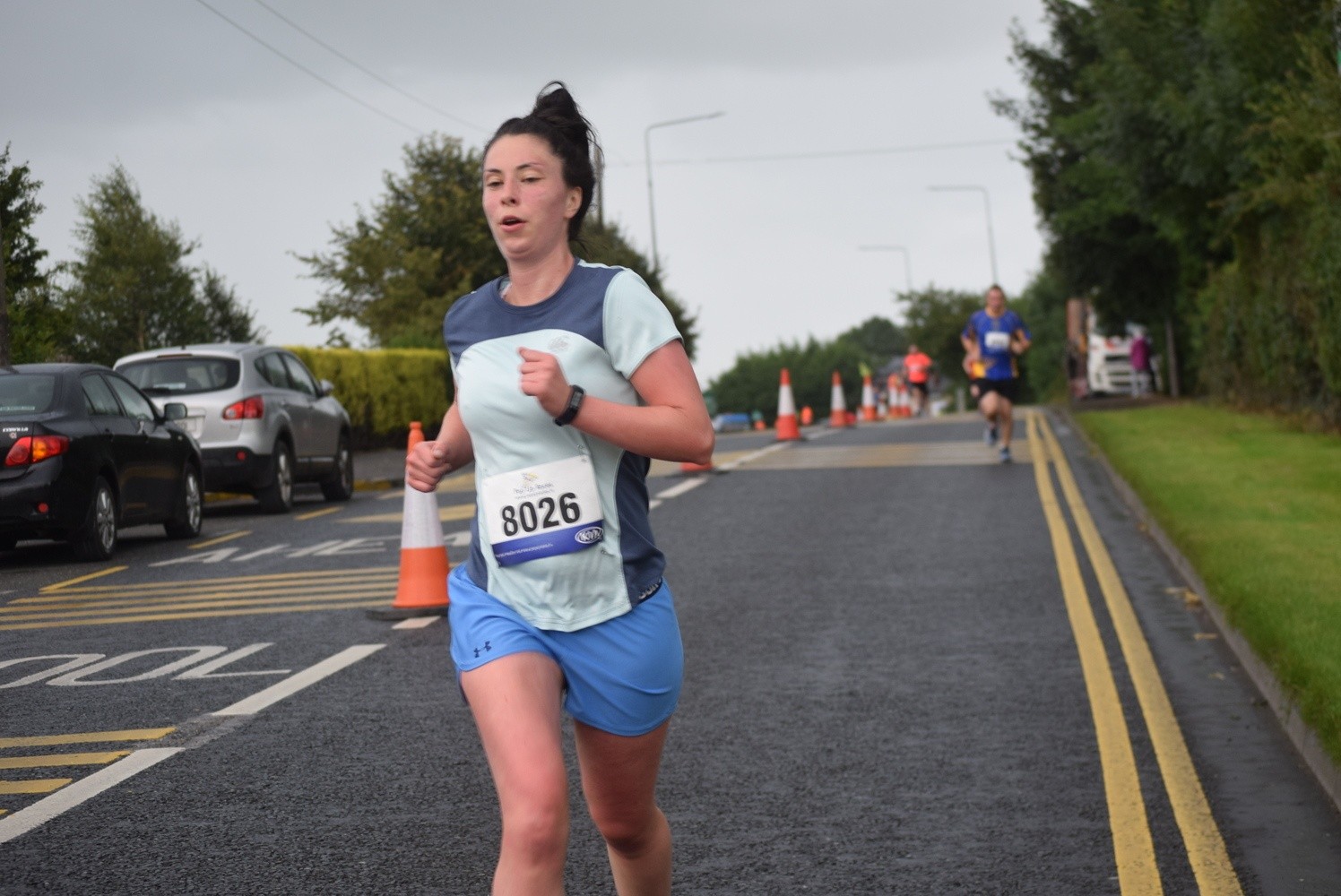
[770,369,807,442]
[367,420,451,619]
[858,375,887,423]
[887,375,912,420]
[661,461,730,480]
[826,370,855,429]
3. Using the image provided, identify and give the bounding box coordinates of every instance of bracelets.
[554,385,584,429]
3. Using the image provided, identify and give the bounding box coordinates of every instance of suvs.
[114,344,354,514]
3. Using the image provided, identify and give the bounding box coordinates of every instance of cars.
[712,412,751,433]
[0,361,204,564]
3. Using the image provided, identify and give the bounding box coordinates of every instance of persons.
[404,83,715,896]
[904,285,1154,463]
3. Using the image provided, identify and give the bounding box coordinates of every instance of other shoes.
[1000,448,1011,463]
[985,429,996,444]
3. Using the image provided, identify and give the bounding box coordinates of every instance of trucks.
[1066,299,1165,402]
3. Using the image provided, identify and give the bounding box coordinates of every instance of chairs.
[178,378,199,389]
